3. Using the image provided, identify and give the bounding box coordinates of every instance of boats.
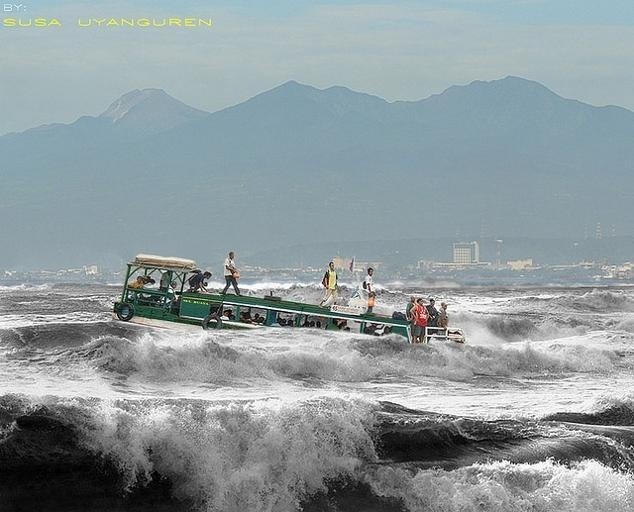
[113,252,466,357]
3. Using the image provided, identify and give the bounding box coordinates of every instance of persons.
[189,271,212,294]
[320,261,339,309]
[222,251,241,296]
[366,291,376,312]
[209,296,450,345]
[158,270,173,292]
[358,267,376,314]
[130,275,156,303]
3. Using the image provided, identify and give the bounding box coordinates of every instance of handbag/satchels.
[232,270,240,279]
[368,296,374,307]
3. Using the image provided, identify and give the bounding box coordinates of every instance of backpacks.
[321,271,329,289]
[363,280,367,290]
